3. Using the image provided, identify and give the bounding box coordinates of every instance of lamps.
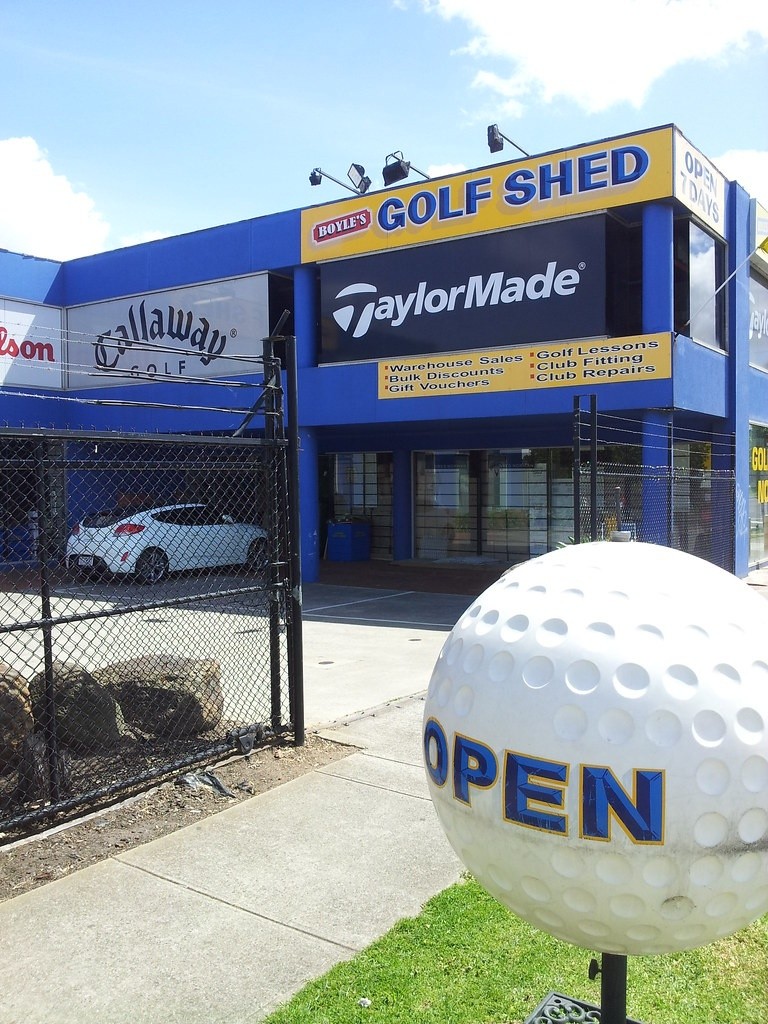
[488,124,504,153]
[348,162,372,193]
[383,150,410,186]
[309,168,323,185]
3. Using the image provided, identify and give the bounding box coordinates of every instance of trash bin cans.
[325,518,374,562]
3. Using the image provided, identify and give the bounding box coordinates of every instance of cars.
[66,503,268,585]
